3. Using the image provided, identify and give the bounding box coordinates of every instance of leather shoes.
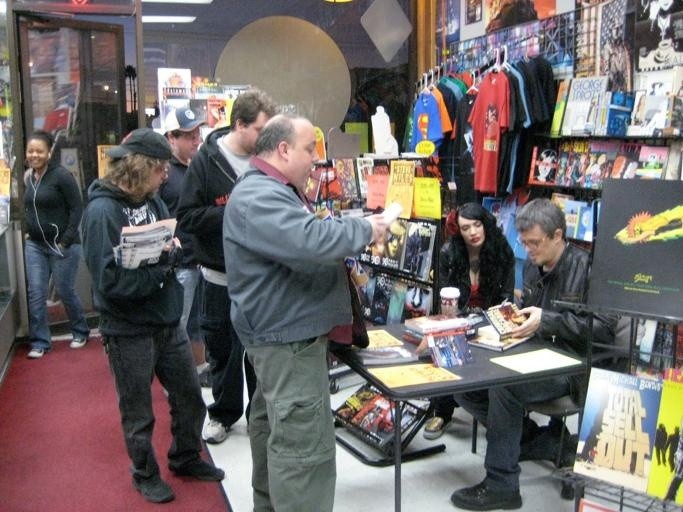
[451,482,523,511]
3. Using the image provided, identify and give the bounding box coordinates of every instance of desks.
[327,317,588,512]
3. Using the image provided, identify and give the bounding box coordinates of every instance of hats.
[165,107,209,134]
[104,127,173,161]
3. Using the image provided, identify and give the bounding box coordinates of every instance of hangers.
[462,68,482,94]
[416,54,461,92]
[482,34,535,74]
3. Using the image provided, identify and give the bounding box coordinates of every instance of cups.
[440,287,460,315]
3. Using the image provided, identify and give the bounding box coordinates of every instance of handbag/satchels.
[347,276,371,350]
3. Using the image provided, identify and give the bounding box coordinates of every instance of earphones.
[48,152,52,159]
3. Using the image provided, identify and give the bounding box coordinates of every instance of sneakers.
[27,348,45,359]
[198,368,212,388]
[168,457,225,482]
[132,474,176,505]
[201,418,228,445]
[70,338,87,349]
[423,414,454,440]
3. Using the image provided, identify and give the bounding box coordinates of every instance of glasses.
[521,233,548,248]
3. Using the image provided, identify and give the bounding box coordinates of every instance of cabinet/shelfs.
[313,155,446,326]
[520,133,683,374]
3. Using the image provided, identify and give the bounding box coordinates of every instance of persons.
[176,89,278,443]
[21,131,90,358]
[161,108,203,398]
[450,201,618,511]
[424,201,516,439]
[79,128,226,499]
[223,114,384,512]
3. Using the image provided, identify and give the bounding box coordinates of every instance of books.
[309,154,443,327]
[334,382,429,448]
[532,64,683,262]
[113,219,179,270]
[352,303,534,369]
